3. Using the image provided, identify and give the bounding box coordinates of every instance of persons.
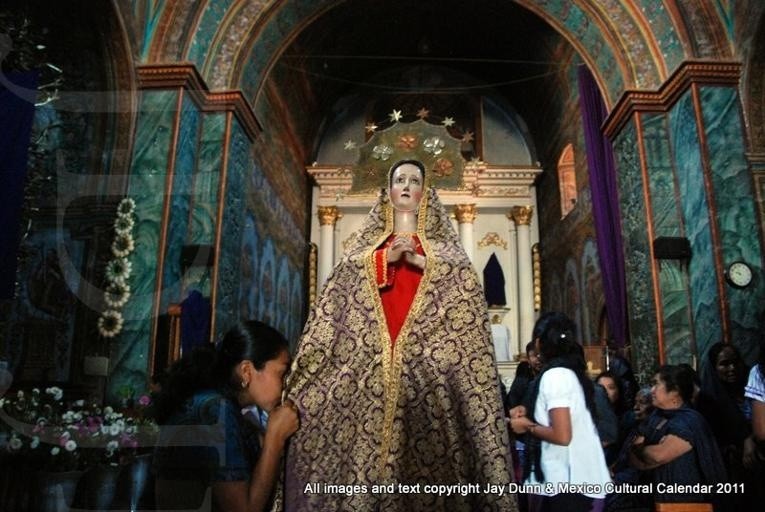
[279,106,523,511]
[146,362,190,427]
[152,318,303,512]
[497,305,764,510]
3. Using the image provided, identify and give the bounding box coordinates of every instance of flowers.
[0,384,155,469]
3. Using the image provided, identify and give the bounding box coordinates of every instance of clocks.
[726,261,753,290]
